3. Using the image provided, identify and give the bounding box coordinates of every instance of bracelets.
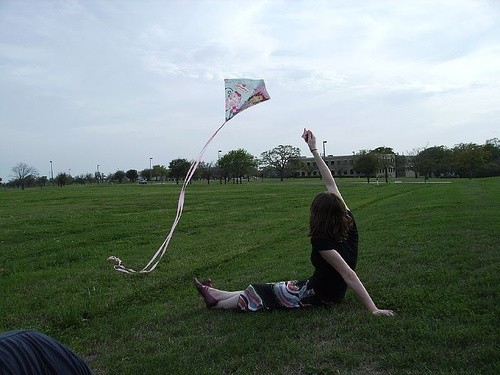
[310,149,318,152]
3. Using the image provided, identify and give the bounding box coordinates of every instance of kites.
[106,78,271,274]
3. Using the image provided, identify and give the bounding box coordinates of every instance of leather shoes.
[202,278,213,309]
[193,277,218,310]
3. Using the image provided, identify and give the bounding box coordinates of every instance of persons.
[1,330,95,374]
[194,127,399,317]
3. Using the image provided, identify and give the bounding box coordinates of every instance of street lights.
[97,164,101,173]
[323,140,327,157]
[218,150,222,160]
[150,157,153,177]
[50,160,53,177]
